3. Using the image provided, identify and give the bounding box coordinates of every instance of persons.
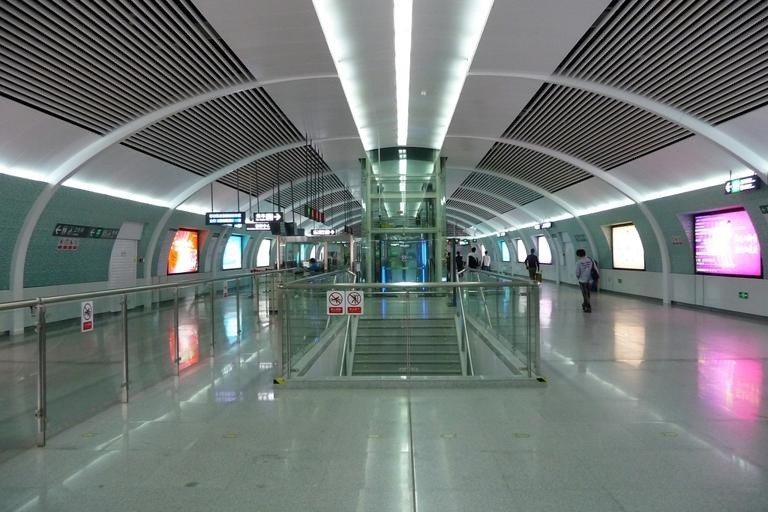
[480,250,491,272]
[445,251,451,279]
[456,251,464,277]
[575,248,599,312]
[467,246,479,269]
[524,248,539,280]
[305,258,323,272]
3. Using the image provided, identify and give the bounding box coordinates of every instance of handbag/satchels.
[536,272,542,282]
[591,265,600,280]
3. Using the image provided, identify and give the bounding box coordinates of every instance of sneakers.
[583,308,591,313]
[581,303,586,308]
